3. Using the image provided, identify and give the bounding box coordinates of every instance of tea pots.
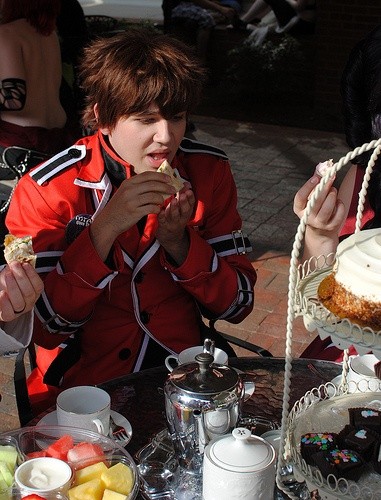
[157,336,246,465]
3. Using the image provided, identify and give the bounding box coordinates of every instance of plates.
[0,426,138,500]
[33,410,132,455]
[327,372,349,398]
[231,367,255,402]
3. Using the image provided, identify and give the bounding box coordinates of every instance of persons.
[292,25,381,375]
[162,0,317,74]
[0,0,66,151]
[0,246,44,358]
[4,27,258,415]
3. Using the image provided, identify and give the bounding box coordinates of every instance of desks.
[18,355,343,500]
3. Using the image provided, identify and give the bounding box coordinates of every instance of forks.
[94,384,129,440]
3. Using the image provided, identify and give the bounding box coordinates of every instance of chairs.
[3,144,273,429]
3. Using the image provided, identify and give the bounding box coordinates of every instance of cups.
[14,457,72,499]
[348,353,381,393]
[165,345,228,373]
[202,427,276,500]
[56,386,111,441]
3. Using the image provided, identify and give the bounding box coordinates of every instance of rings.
[14,301,26,314]
